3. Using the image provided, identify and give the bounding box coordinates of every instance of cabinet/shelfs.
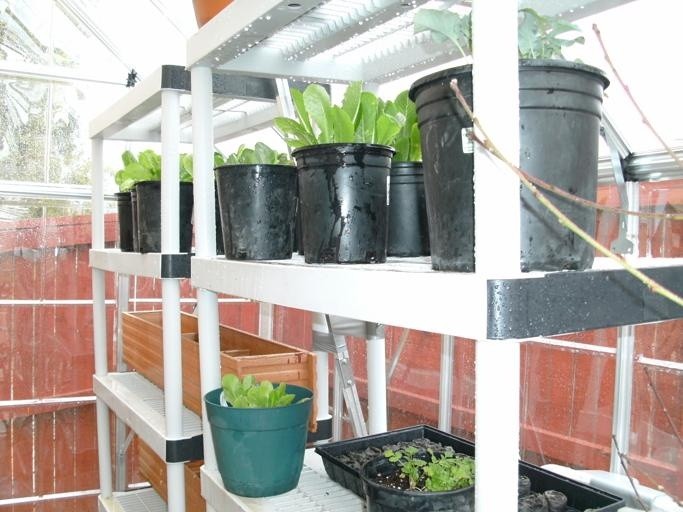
[198,0,682,512]
[88,79,320,512]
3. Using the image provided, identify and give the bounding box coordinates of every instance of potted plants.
[406,5,611,275]
[113,148,219,254]
[213,145,303,259]
[273,82,432,266]
[203,374,314,498]
[361,445,475,512]
